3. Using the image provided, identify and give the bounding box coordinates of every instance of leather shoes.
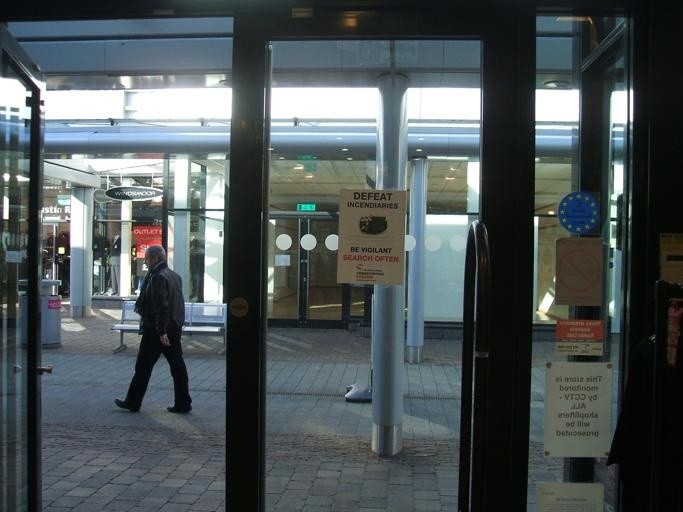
[167,403,192,413]
[114,399,140,412]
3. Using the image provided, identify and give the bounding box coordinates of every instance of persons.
[110,235,121,296]
[93,225,110,295]
[113,245,193,414]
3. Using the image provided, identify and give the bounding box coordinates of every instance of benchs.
[110,298,225,360]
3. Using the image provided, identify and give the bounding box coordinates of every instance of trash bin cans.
[15,278,63,351]
